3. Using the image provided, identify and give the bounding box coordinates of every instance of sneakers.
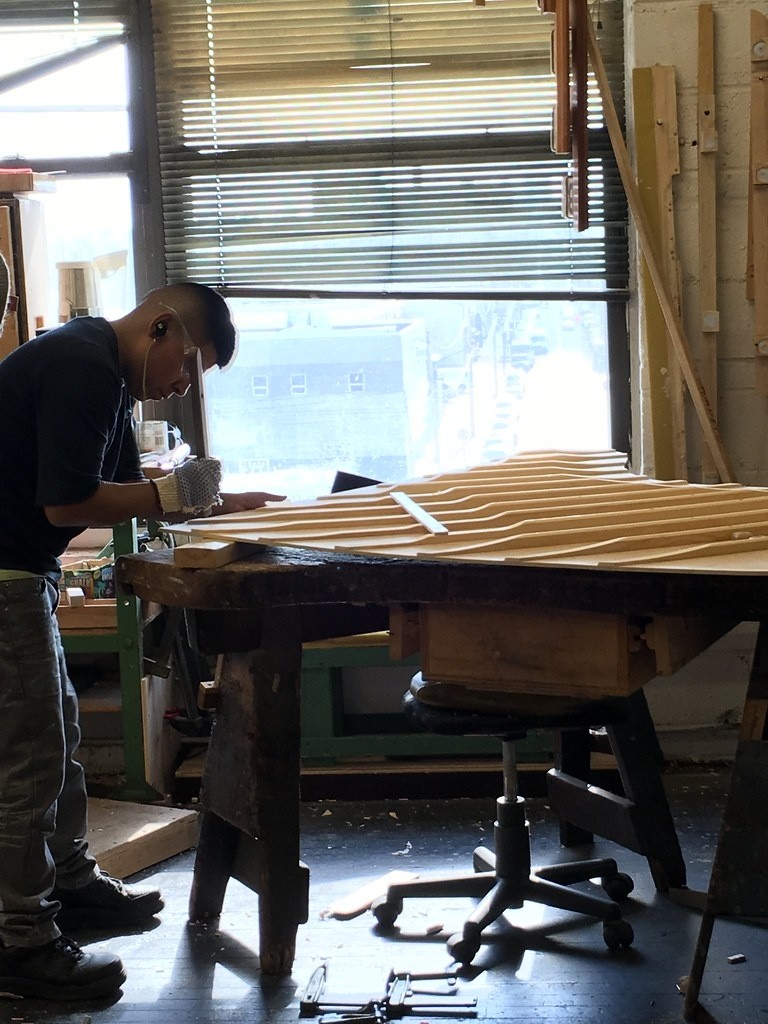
[0,934,126,1000]
[46,870,164,928]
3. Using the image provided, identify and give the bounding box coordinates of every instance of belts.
[0,569,46,581]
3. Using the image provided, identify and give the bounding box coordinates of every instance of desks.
[122,538,768,965]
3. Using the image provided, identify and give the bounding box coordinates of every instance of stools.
[372,686,647,953]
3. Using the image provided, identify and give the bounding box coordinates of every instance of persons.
[0,282,287,1001]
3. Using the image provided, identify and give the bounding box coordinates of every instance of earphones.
[155,321,167,337]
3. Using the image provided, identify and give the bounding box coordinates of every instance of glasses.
[159,301,195,379]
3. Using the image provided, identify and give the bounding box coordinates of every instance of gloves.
[150,457,222,515]
